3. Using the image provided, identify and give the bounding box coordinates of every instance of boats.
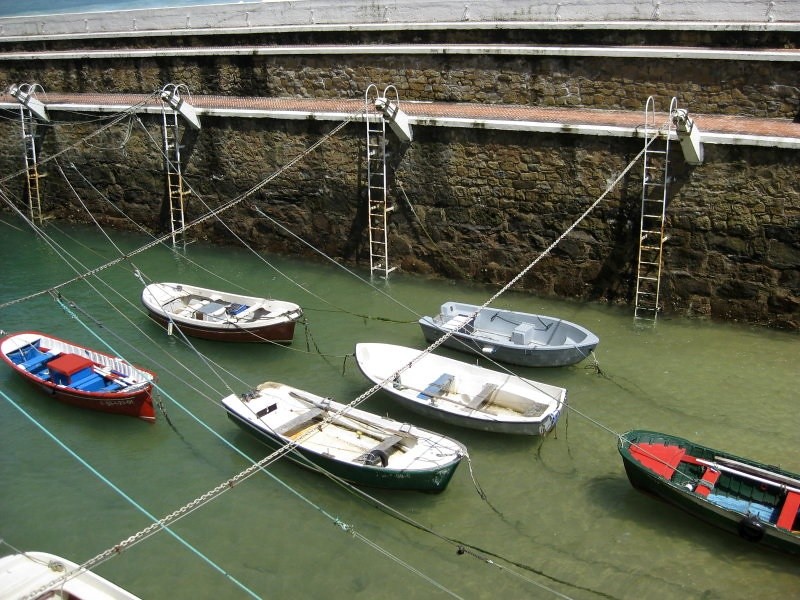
[217,382,467,495]
[143,281,303,347]
[354,342,567,436]
[1,550,140,600]
[616,431,800,545]
[418,300,599,369]
[0,333,159,423]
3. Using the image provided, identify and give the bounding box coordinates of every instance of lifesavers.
[365,449,387,471]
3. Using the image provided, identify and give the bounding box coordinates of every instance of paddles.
[374,374,498,416]
[695,458,800,493]
[92,364,136,388]
[288,392,414,439]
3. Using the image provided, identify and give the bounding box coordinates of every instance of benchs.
[466,383,498,410]
[775,491,800,532]
[415,372,456,402]
[98,381,127,393]
[440,312,483,332]
[510,321,536,345]
[351,430,409,465]
[67,372,107,392]
[694,466,722,499]
[273,406,326,435]
[16,349,61,371]
[178,295,221,318]
[227,302,265,323]
